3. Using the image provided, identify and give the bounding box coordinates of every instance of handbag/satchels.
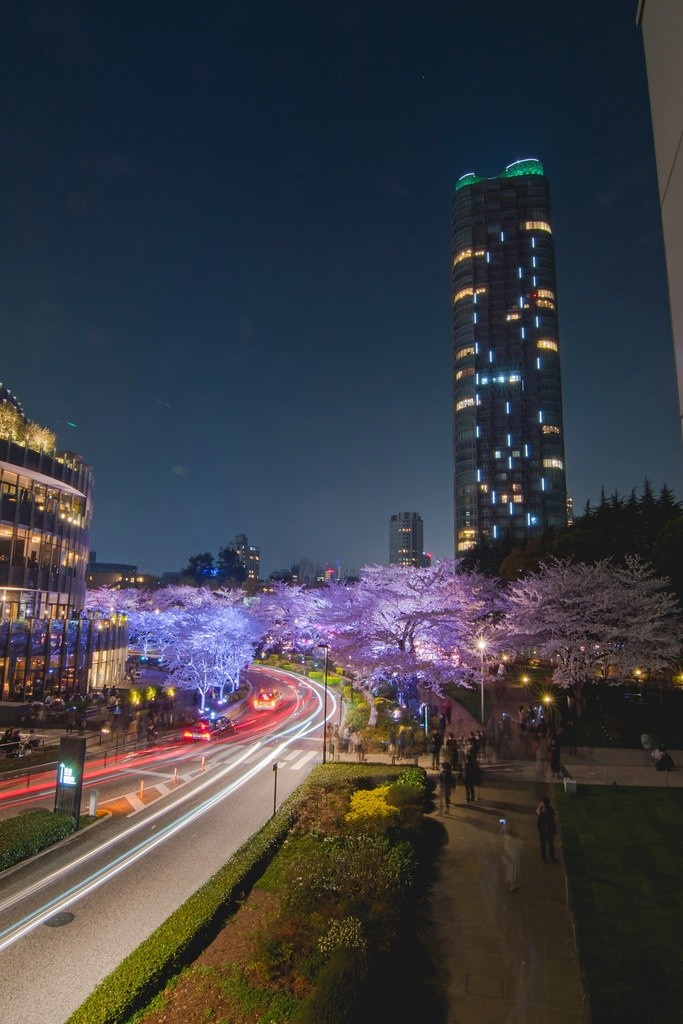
[337,745,339,748]
[427,744,435,752]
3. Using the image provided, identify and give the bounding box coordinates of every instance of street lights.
[315,643,328,765]
[478,642,486,725]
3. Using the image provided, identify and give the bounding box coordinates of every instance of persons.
[0,684,157,758]
[536,796,557,863]
[323,693,673,803]
[434,762,453,816]
[502,823,530,893]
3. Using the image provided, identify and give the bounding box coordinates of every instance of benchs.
[29,688,129,720]
[0,737,44,761]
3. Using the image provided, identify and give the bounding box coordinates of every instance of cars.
[134,671,142,679]
[253,688,283,711]
[182,715,239,741]
